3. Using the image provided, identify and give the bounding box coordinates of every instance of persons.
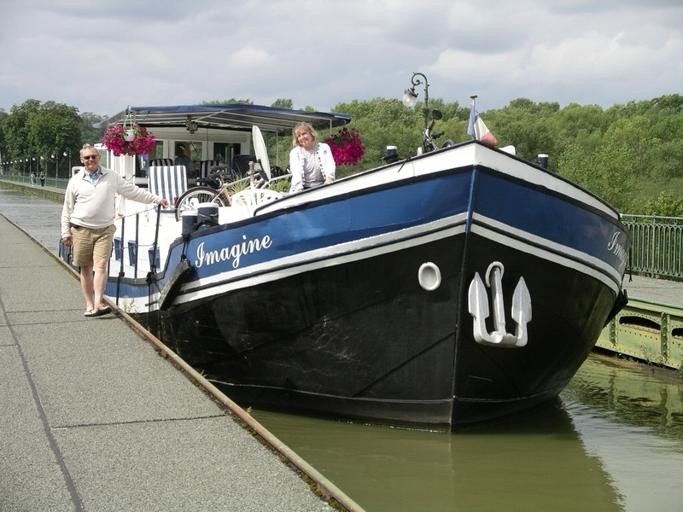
[60,144,169,316]
[39,171,46,186]
[285,120,336,192]
[30,171,36,185]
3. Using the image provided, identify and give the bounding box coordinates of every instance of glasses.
[81,154,97,160]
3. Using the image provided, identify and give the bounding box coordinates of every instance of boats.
[243,397,626,511]
[58,94,631,435]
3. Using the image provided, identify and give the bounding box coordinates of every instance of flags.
[466,104,500,150]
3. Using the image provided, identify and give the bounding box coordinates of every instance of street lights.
[0,146,70,189]
[402,72,429,153]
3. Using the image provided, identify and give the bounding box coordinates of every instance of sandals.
[84,306,93,317]
[91,303,112,317]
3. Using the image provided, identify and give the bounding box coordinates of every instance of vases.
[123,129,135,141]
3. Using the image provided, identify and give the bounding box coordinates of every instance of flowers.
[100,123,156,156]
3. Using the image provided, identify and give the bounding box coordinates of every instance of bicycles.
[410,109,454,156]
[174,158,291,222]
